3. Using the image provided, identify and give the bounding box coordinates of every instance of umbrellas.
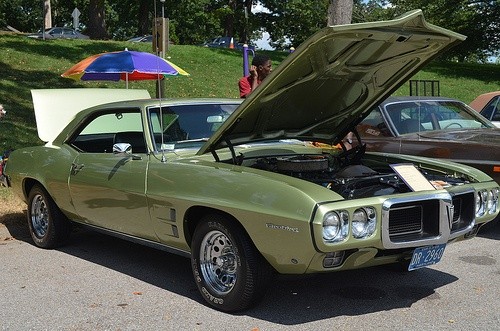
[61,48,190,89]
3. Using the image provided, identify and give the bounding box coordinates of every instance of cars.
[419,91,500,130]
[346,97,500,185]
[5,9,500,314]
[128,35,174,45]
[203,37,265,53]
[26,27,90,40]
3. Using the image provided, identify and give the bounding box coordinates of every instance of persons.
[238,54,272,99]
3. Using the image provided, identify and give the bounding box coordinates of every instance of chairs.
[398,119,426,134]
[112,132,148,153]
